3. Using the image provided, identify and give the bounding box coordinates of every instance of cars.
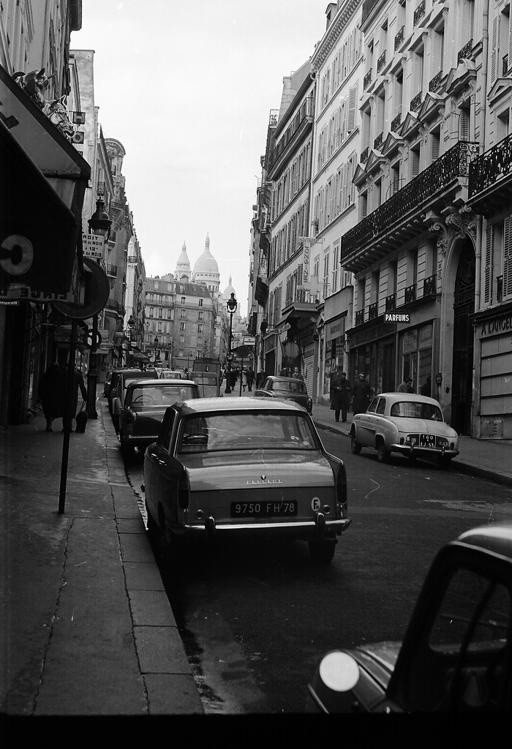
[304,521,512,713]
[349,392,459,464]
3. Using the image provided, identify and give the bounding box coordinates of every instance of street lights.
[88,198,112,420]
[225,293,237,394]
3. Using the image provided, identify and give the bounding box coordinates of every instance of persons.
[60,358,88,433]
[38,358,63,433]
[328,371,431,423]
[229,366,304,393]
